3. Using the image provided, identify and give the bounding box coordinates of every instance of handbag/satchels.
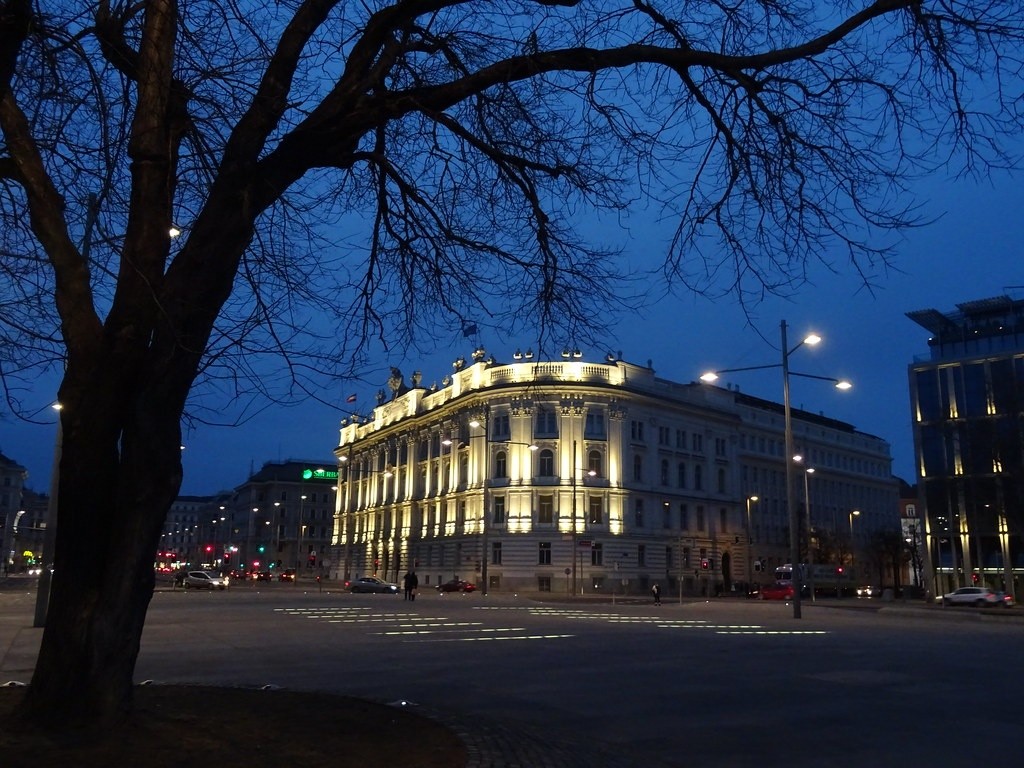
[411,589,418,595]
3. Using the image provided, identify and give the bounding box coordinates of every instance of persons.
[404,570,418,601]
[817,583,824,599]
[651,581,662,606]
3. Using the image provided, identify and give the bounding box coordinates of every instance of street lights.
[315,445,394,590]
[442,402,539,595]
[848,511,861,595]
[700,319,852,620]
[747,495,758,597]
[793,455,816,603]
[571,440,597,597]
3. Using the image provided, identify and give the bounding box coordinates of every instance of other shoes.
[655,604,657,606]
[659,604,660,606]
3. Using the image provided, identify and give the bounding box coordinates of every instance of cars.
[228,568,296,582]
[183,570,226,591]
[345,576,402,594]
[942,586,1016,609]
[754,584,796,601]
[856,586,881,597]
[436,579,477,593]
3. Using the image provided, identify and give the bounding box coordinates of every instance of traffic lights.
[224,552,231,564]
[702,559,710,570]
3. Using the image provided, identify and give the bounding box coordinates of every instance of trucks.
[772,563,839,599]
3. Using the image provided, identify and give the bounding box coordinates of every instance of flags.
[463,324,477,337]
